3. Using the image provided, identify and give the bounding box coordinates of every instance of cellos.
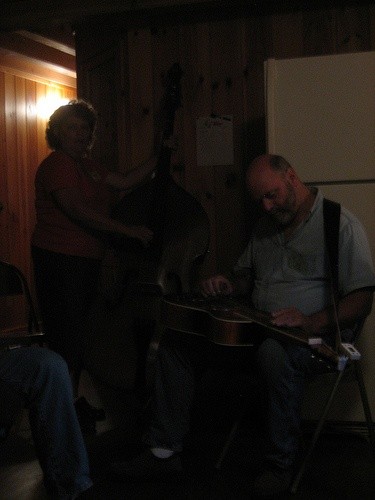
[80,62,211,392]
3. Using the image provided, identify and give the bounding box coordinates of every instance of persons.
[0,347,97,500]
[30,99,152,398]
[106,154,375,500]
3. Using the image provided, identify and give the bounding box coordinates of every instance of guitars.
[161,293,350,370]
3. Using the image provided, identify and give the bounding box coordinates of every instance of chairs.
[0,261,46,348]
[215,317,375,492]
